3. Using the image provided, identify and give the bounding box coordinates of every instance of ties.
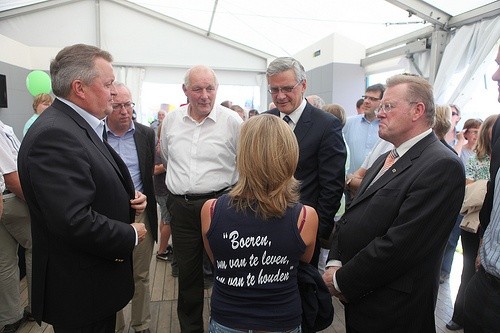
[283,115,290,123]
[365,149,399,191]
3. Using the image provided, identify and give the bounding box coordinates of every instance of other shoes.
[205,278,216,289]
[440,270,450,284]
[0,306,30,333]
[446,320,463,330]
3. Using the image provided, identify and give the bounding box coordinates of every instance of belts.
[180,185,236,201]
[3,190,11,195]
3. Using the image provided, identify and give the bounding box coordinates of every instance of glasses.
[378,103,417,114]
[452,112,458,116]
[362,96,381,102]
[466,131,478,135]
[268,81,302,94]
[113,102,135,111]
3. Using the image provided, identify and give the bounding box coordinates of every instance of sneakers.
[167,245,173,254]
[156,250,174,261]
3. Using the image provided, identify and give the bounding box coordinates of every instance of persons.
[200,113,318,333]
[23,93,55,138]
[18,43,147,333]
[259,55,347,270]
[102,83,159,333]
[159,66,246,333]
[131,83,500,333]
[0,122,35,333]
[321,74,466,333]
[462,44,500,333]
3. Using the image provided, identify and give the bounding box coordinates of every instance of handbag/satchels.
[297,261,334,333]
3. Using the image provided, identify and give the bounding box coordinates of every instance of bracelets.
[346,176,354,188]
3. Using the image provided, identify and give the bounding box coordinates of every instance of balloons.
[25,70,53,97]
